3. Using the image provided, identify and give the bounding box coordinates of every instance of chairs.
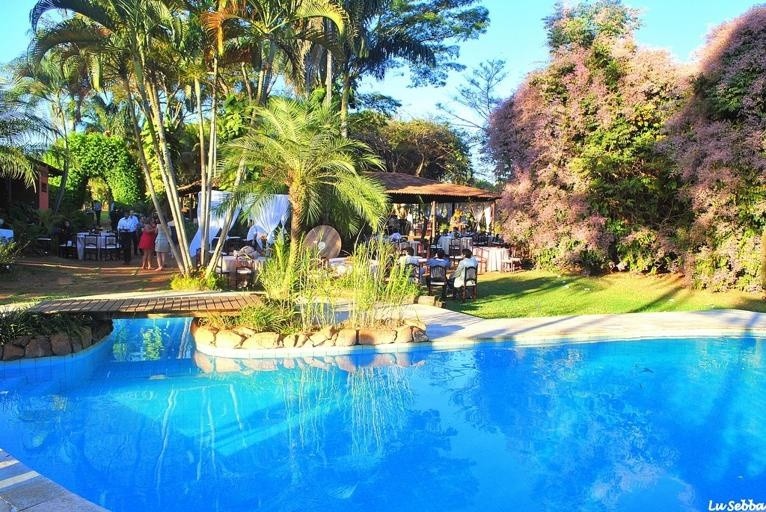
[501,248,522,273]
[62,234,120,261]
[402,263,477,301]
[195,248,253,288]
[450,236,487,274]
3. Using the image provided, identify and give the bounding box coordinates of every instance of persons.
[59,220,73,258]
[94,200,172,272]
[247,219,257,251]
[388,216,493,300]
